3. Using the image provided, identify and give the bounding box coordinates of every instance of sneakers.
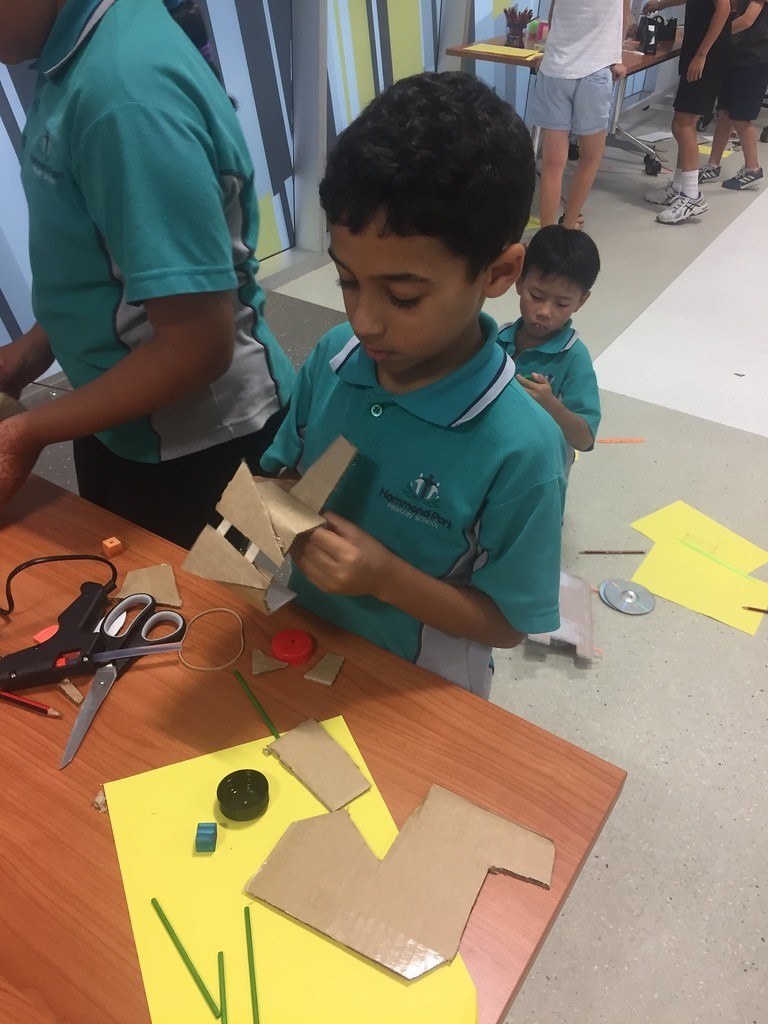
[722,165,763,190]
[697,162,721,183]
[656,187,709,224]
[644,177,684,205]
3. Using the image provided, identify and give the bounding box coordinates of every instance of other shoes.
[560,212,584,231]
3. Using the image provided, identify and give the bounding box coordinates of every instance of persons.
[638,0,768,222]
[492,225,600,463]
[523,0,631,230]
[0,0,300,549]
[258,69,569,699]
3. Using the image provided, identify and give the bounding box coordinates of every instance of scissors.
[59,594,186,771]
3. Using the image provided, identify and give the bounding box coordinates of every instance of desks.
[444,34,546,73]
[569,22,686,174]
[0,469,628,1024]
